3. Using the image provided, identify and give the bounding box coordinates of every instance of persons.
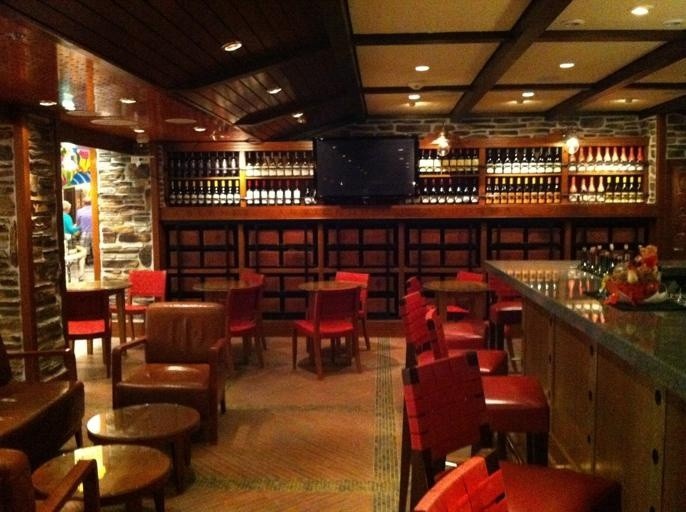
[74,195,93,282]
[62,200,81,258]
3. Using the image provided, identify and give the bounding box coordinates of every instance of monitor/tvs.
[313,134,419,204]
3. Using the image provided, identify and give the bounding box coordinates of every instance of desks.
[81,400,207,497]
[67,280,133,358]
[30,443,171,512]
[295,279,368,376]
[190,281,263,365]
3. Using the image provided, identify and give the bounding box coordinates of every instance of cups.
[657,282,681,303]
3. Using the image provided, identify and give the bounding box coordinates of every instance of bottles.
[580,242,632,274]
[169,153,319,206]
[405,146,645,202]
[506,269,605,324]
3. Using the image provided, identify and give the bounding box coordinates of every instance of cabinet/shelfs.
[482,136,564,204]
[400,137,482,206]
[155,141,240,205]
[242,141,318,206]
[564,138,647,202]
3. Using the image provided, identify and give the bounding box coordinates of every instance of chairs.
[290,287,362,377]
[0,442,105,512]
[217,287,268,372]
[329,270,374,352]
[394,268,624,512]
[108,268,169,343]
[108,302,237,445]
[64,288,115,364]
[235,268,272,353]
[0,337,85,456]
